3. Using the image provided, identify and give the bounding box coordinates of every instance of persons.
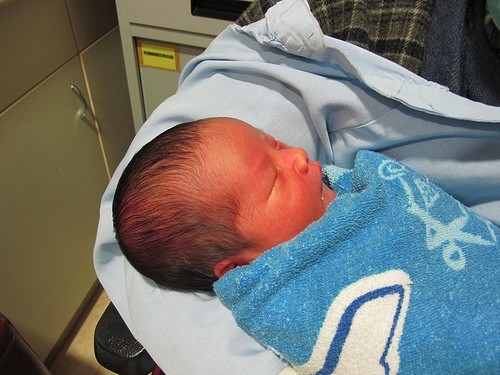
[161,0,500,375]
[112,117,500,375]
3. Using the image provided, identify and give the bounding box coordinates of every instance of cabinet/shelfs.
[0,0,136,375]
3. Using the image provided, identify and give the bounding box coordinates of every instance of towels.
[213,149,500,375]
